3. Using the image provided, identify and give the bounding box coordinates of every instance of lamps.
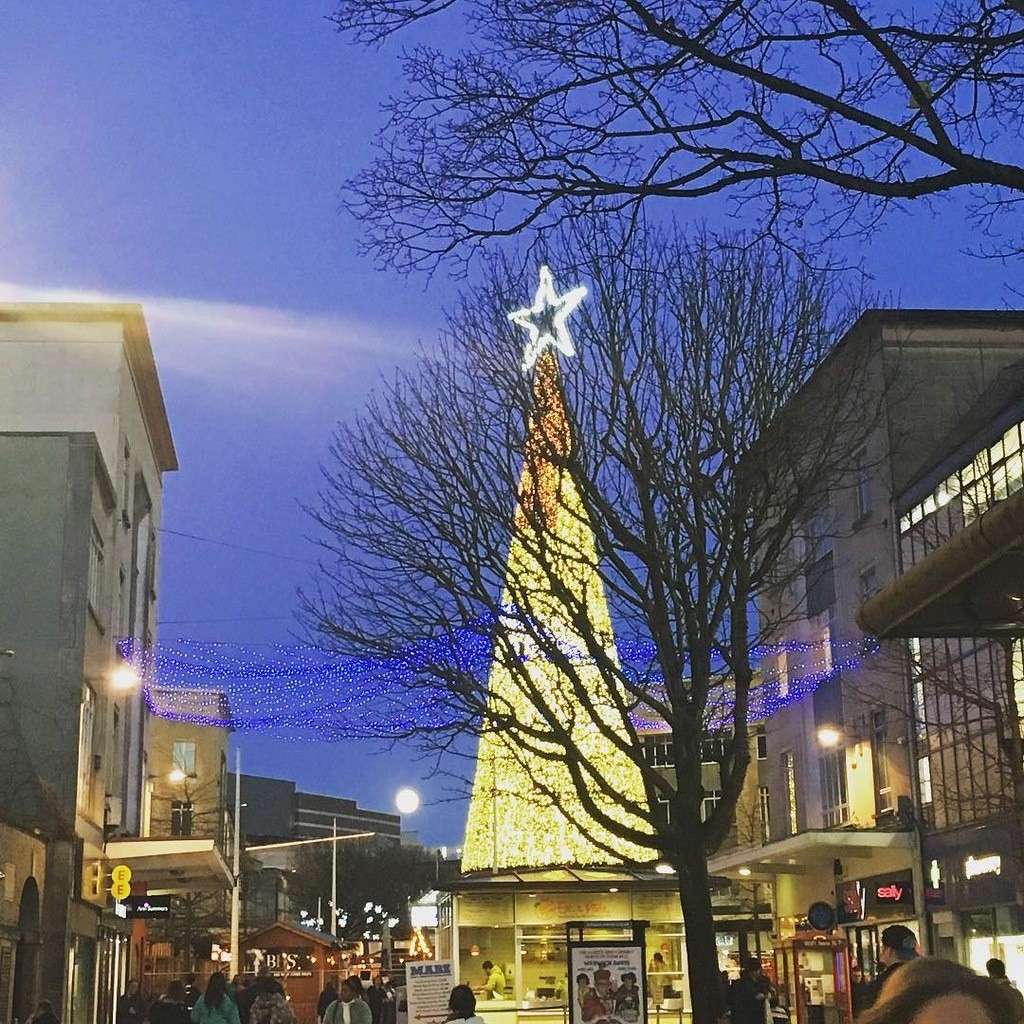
[818,726,862,751]
[149,770,185,782]
[471,943,480,956]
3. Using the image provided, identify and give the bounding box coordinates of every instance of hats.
[882,925,919,959]
[621,972,636,983]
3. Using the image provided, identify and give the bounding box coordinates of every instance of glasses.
[596,983,609,988]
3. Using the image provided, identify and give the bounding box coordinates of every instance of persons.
[27,999,59,1024]
[985,959,1024,1024]
[443,985,487,1024]
[149,965,296,1024]
[874,924,918,989]
[577,968,640,1022]
[474,961,506,997]
[861,955,1017,1024]
[117,980,146,1024]
[730,958,771,1024]
[317,976,387,1024]
[653,953,674,998]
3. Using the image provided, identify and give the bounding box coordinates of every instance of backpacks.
[249,991,297,1024]
